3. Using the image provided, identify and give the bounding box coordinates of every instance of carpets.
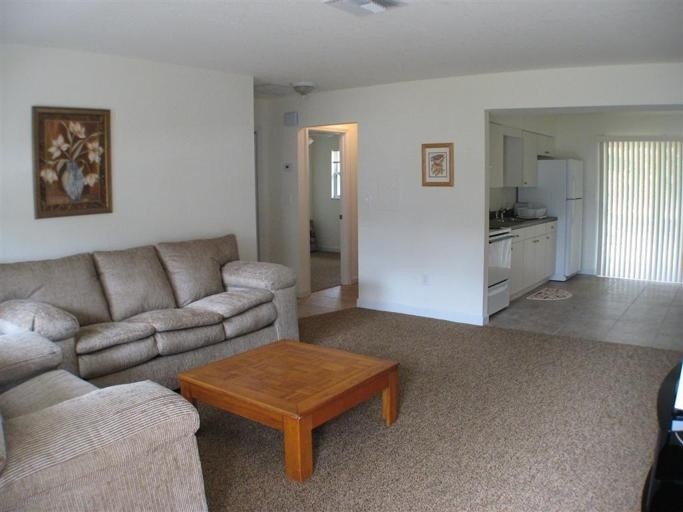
[525,285,574,302]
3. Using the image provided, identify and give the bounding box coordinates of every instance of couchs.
[2,233,300,386]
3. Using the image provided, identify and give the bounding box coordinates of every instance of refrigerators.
[518,159,583,281]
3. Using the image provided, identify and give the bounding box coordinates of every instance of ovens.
[488,229,512,315]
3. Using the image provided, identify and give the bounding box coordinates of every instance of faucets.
[493,207,503,218]
[500,209,507,222]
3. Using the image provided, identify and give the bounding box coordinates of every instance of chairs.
[1,313,234,509]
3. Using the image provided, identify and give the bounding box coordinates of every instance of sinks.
[490,217,526,227]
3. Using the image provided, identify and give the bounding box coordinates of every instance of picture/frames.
[29,103,114,220]
[420,143,455,187]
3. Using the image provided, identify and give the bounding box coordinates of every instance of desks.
[171,335,401,482]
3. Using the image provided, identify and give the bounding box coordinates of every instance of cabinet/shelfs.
[511,216,558,302]
[485,124,555,189]
